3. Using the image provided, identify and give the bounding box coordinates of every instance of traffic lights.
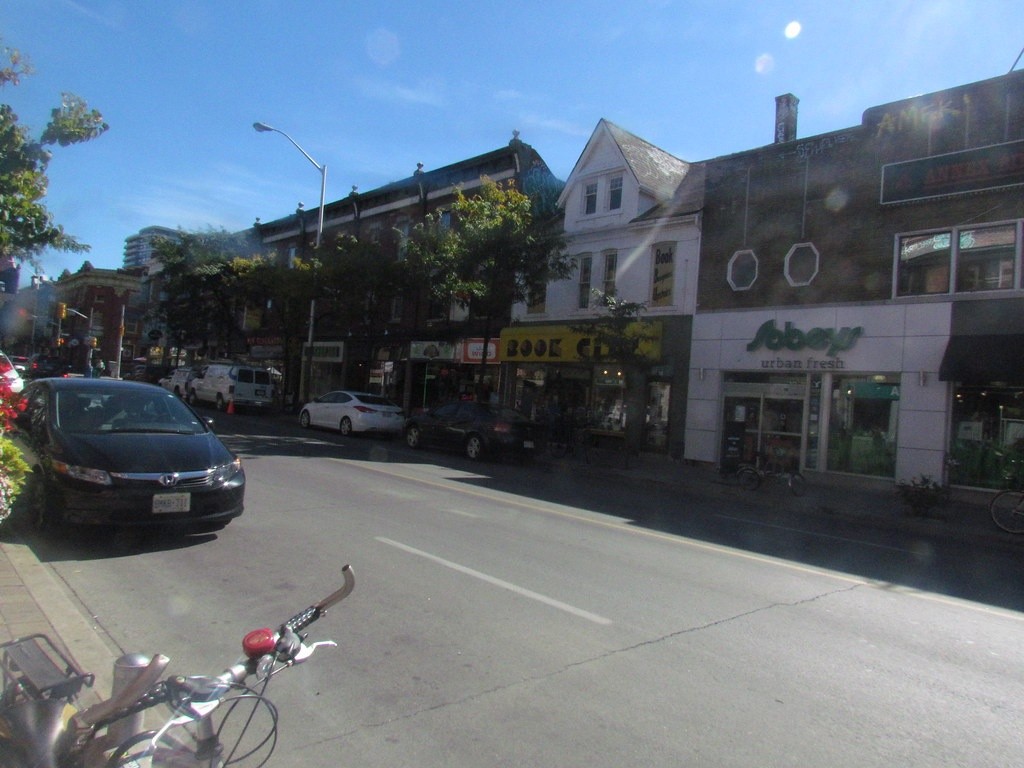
[57,302,67,319]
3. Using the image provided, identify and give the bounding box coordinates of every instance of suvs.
[156,367,200,402]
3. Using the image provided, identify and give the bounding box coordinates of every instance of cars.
[7,354,30,377]
[5,375,248,541]
[24,352,69,380]
[400,399,547,464]
[0,349,24,394]
[297,389,406,440]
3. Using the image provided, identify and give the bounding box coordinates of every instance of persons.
[94,358,106,378]
[461,392,472,402]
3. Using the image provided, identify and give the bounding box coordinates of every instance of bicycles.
[989,486,1024,535]
[1,565,356,767]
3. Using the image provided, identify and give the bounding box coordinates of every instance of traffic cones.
[227,399,235,416]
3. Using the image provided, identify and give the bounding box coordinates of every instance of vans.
[186,362,275,415]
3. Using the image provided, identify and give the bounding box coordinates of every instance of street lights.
[254,120,328,417]
